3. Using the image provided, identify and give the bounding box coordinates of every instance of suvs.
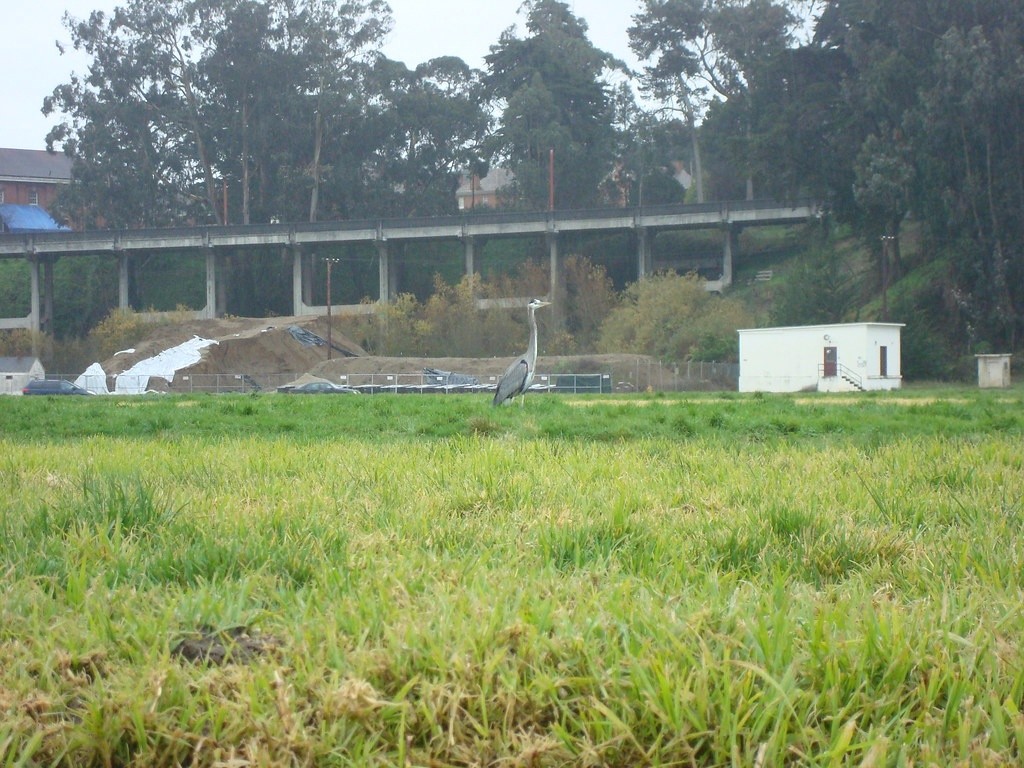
[22,379,99,397]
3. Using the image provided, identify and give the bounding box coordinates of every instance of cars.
[288,381,363,395]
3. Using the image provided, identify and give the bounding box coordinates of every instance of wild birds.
[490,297,553,408]
